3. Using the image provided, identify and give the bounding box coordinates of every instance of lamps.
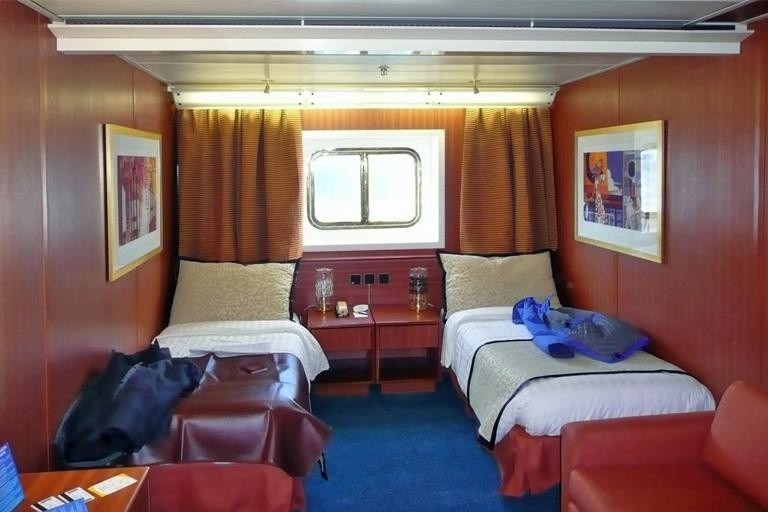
[408,265,428,312]
[312,268,336,314]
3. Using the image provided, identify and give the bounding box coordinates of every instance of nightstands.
[305,302,441,396]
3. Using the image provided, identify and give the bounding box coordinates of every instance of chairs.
[556,378,767,509]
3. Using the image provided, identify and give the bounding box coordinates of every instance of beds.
[440,307,711,492]
[62,306,302,465]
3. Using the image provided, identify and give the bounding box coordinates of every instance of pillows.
[165,258,299,324]
[435,245,563,310]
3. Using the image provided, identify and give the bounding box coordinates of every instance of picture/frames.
[571,119,667,265]
[103,122,164,284]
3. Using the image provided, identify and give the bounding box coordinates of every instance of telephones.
[335,300,348,317]
